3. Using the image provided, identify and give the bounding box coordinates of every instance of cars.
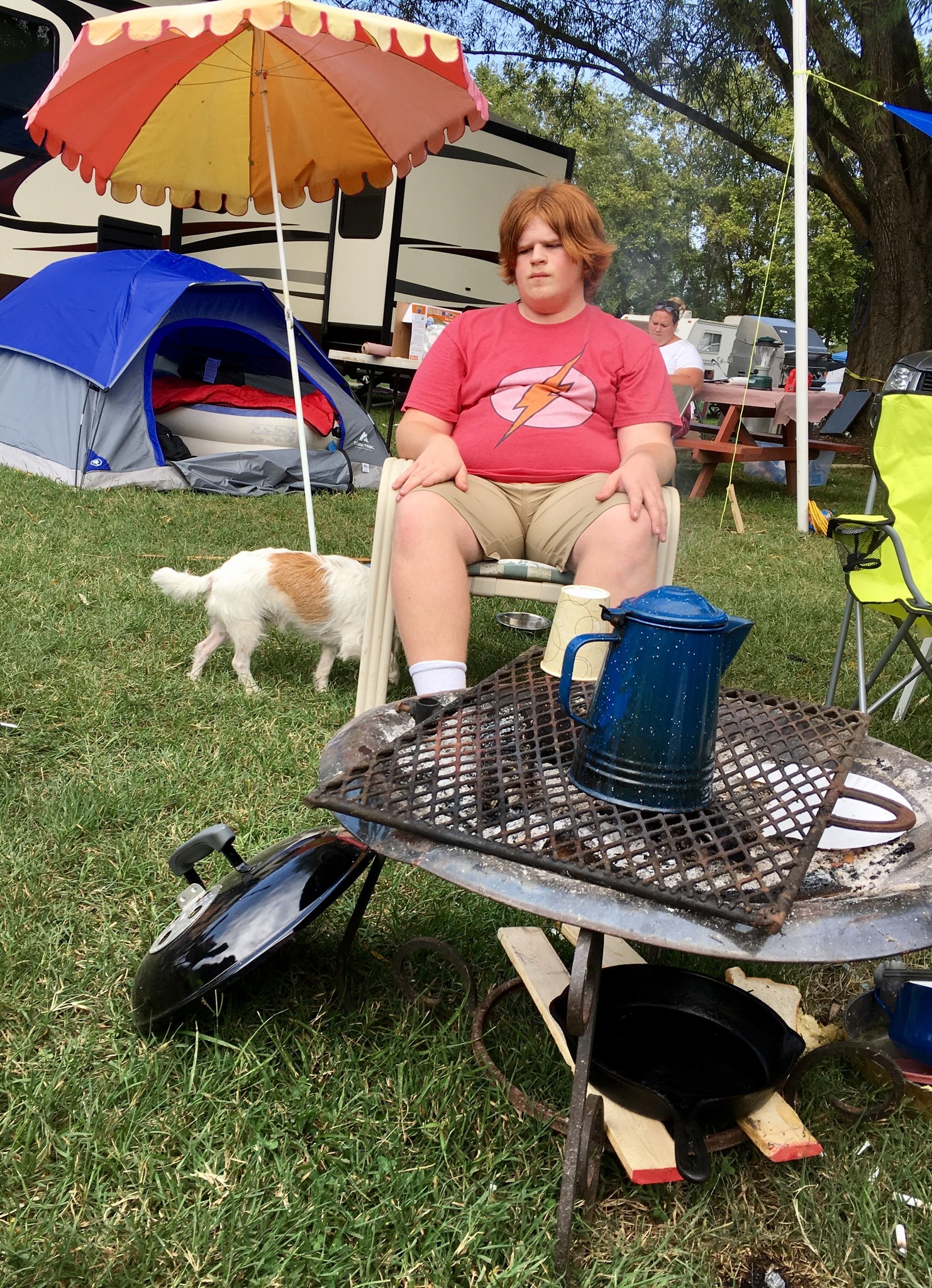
[870,350,932,433]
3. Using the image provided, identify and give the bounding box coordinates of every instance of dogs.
[150,546,401,694]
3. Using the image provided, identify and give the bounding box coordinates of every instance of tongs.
[873,960,932,992]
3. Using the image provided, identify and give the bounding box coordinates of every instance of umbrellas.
[20,1,491,572]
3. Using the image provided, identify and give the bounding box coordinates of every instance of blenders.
[744,336,784,391]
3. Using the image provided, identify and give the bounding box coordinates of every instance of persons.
[634,294,703,439]
[669,295,685,315]
[387,178,684,695]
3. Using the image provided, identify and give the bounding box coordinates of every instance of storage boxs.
[390,300,464,362]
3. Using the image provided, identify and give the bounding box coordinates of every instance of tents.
[0,249,395,498]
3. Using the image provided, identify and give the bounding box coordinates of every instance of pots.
[549,964,806,1183]
[843,986,910,1058]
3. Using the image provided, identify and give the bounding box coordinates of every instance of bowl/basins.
[495,612,551,633]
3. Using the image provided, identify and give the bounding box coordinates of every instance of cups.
[875,980,932,1066]
[539,585,612,681]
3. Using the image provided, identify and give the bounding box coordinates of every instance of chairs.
[825,391,931,734]
[350,455,681,724]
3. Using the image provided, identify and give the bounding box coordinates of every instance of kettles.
[558,586,756,813]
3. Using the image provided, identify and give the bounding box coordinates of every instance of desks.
[327,347,439,450]
[687,380,845,505]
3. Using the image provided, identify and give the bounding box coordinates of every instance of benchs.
[685,419,722,435]
[751,430,864,456]
[675,435,765,455]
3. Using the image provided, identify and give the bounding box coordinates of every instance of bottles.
[809,508,837,526]
